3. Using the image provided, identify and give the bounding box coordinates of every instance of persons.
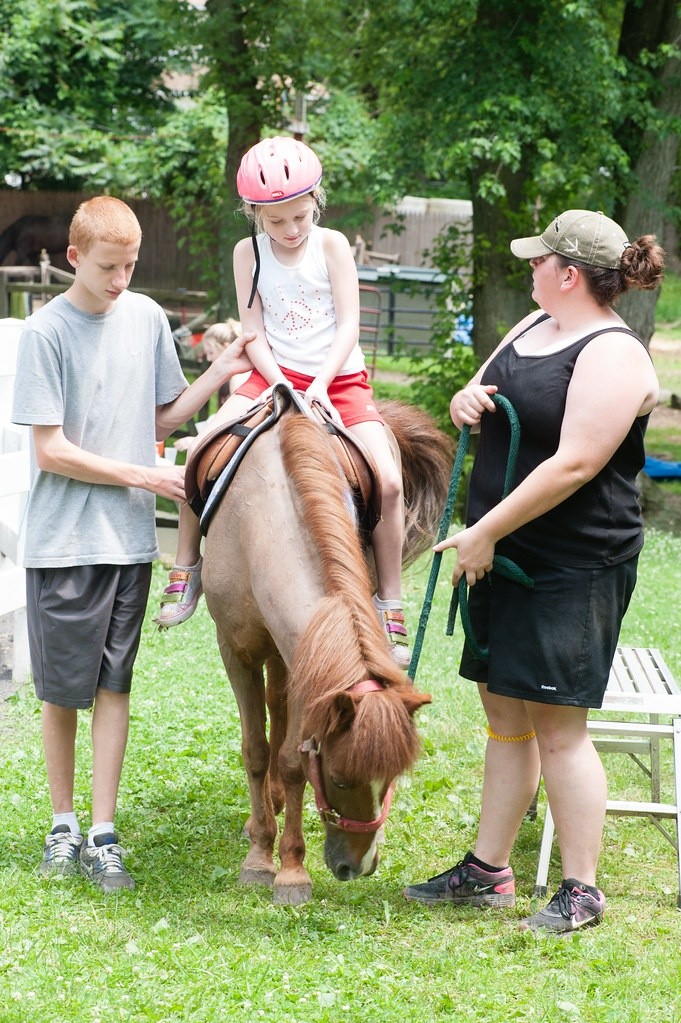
[406,209,666,940]
[156,134,413,670]
[11,195,258,899]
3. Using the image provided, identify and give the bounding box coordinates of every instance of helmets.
[236,136,324,205]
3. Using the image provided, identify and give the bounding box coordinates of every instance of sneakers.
[372,594,413,670]
[515,879,606,937]
[405,859,513,907]
[81,834,135,890]
[44,826,80,879]
[154,555,204,624]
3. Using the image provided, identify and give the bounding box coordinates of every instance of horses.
[194,393,464,906]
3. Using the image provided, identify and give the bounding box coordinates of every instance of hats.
[512,209,629,270]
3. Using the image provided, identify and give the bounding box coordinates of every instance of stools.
[526,641,681,910]
